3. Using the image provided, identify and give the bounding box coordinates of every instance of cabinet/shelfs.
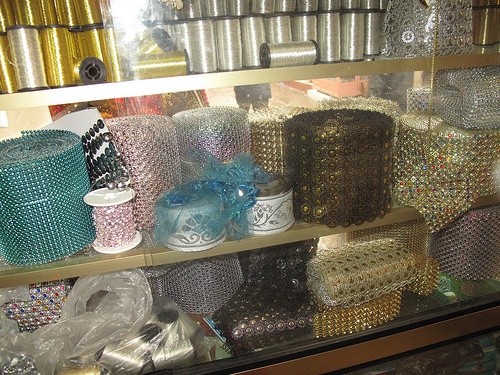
[1,1,500,374]
